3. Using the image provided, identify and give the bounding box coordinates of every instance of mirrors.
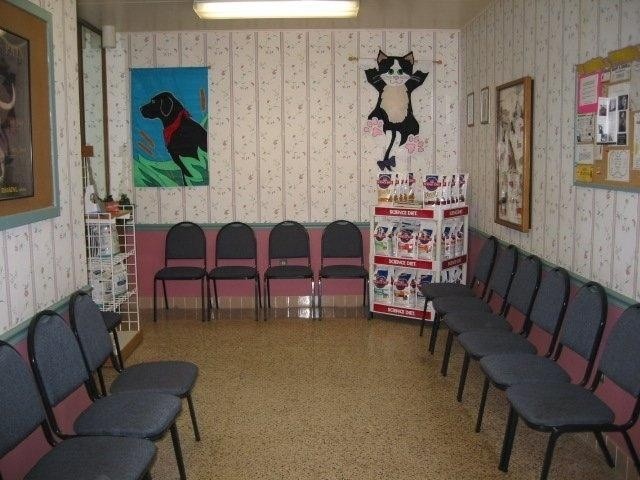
[78,17,111,204]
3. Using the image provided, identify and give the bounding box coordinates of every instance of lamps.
[101,25,116,50]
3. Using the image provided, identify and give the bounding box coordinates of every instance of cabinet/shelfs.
[85,204,143,367]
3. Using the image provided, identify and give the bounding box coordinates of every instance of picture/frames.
[493,75,532,234]
[0,0,62,232]
[466,86,489,127]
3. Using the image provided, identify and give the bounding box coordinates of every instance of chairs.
[1,290,201,480]
[153,220,369,323]
